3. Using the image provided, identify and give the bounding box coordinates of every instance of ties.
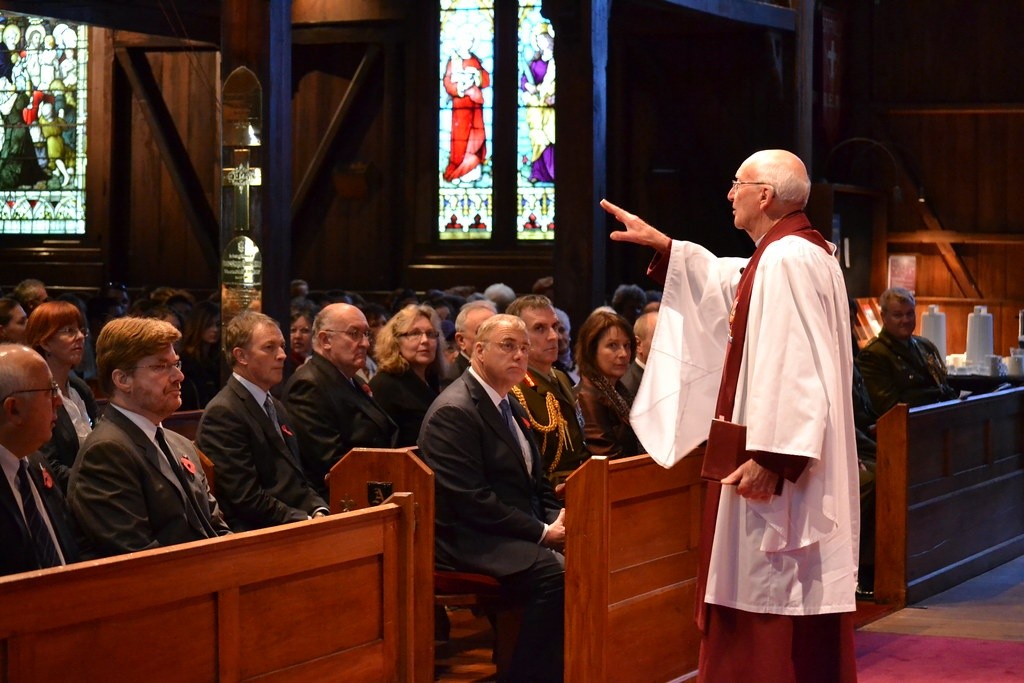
[264,396,285,443]
[908,339,916,353]
[155,428,219,537]
[499,399,522,451]
[16,460,62,569]
[551,376,560,391]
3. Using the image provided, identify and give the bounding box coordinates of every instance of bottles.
[921,304,946,375]
[966,306,992,376]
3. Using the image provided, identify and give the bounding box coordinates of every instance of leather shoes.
[856,570,874,600]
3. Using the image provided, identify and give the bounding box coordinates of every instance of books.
[700,419,784,497]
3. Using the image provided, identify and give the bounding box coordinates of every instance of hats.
[441,319,456,338]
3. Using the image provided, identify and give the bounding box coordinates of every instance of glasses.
[731,179,776,198]
[483,339,533,356]
[398,329,440,340]
[107,281,128,292]
[57,327,88,337]
[1,382,59,406]
[321,328,372,340]
[123,361,182,372]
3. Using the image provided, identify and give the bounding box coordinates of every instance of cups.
[1004,355,1024,376]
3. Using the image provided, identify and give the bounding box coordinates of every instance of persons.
[125,286,223,411]
[289,279,573,382]
[281,303,399,504]
[67,316,234,558]
[0,278,99,492]
[196,312,331,531]
[507,294,592,483]
[0,342,90,575]
[600,149,861,683]
[612,284,663,397]
[417,313,565,683]
[855,287,955,416]
[366,305,445,448]
[848,296,881,600]
[571,311,648,461]
